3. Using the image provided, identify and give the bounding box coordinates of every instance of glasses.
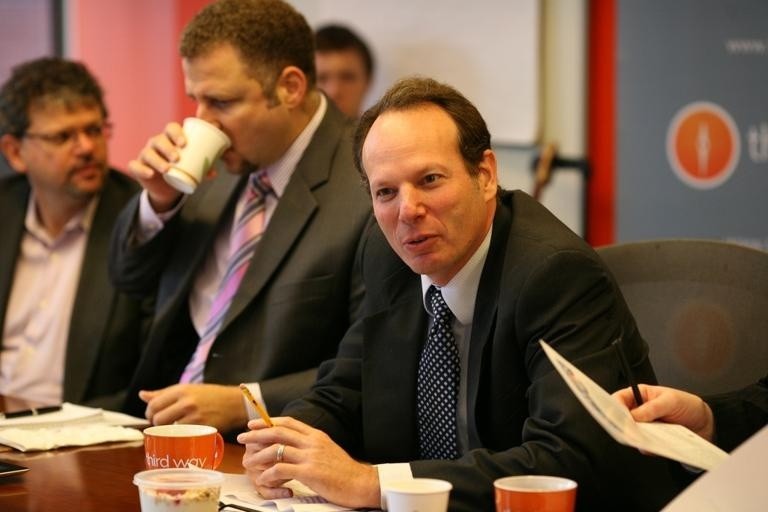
[21,123,113,149]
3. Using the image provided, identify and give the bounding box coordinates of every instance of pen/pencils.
[240,383,274,428]
[5,407,62,419]
[615,341,644,407]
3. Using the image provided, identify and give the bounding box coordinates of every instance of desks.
[0,394,391,511]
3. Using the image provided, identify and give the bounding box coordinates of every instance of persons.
[611,383,767,473]
[235,75,687,511]
[311,23,373,118]
[107,0,369,438]
[0,56,142,414]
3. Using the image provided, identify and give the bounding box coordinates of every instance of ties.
[418,288,459,460]
[179,170,271,387]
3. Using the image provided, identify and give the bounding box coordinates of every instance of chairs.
[586,236,768,511]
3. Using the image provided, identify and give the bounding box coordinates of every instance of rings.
[277,443,287,461]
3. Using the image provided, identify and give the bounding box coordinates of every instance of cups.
[144,423,225,472]
[132,467,225,512]
[494,474,581,512]
[382,476,454,511]
[162,115,234,197]
